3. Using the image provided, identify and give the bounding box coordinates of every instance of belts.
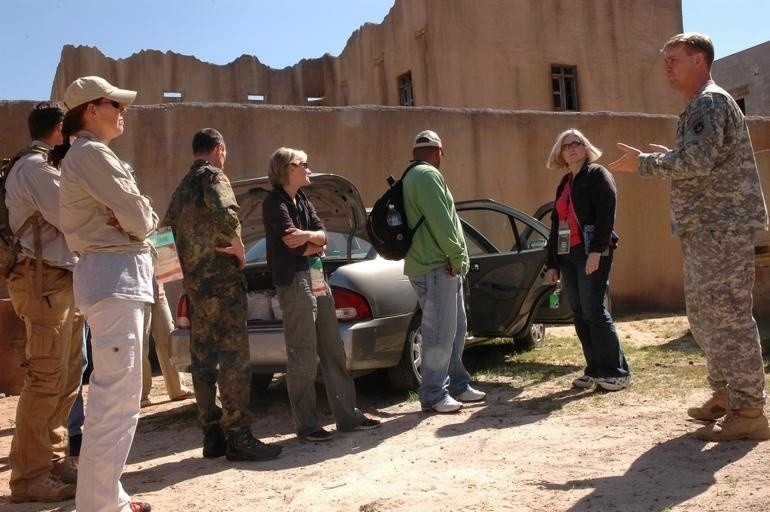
[17,253,63,270]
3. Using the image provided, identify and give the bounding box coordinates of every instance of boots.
[227,430,281,461]
[203,422,227,457]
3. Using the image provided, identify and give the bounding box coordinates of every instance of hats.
[413,130,444,156]
[63,75,138,111]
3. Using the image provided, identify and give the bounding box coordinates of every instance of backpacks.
[365,163,431,261]
[0,146,53,279]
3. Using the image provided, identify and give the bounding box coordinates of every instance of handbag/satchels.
[584,225,610,257]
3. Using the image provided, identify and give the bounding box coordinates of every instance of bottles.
[548,280,562,309]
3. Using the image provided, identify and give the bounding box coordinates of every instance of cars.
[163,157,614,395]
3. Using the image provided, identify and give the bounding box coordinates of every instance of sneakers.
[687,392,730,420]
[353,419,380,430]
[453,385,486,401]
[11,479,77,502]
[130,501,151,512]
[597,377,633,391]
[174,390,196,400]
[297,428,333,441]
[52,460,78,482]
[696,413,770,440]
[422,395,463,413]
[572,374,606,387]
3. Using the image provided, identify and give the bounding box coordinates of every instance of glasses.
[561,140,584,151]
[81,101,120,109]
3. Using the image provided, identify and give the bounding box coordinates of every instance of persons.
[159,127,282,462]
[607,31,769,440]
[263,146,381,442]
[140,285,196,408]
[546,128,634,390]
[5,104,85,503]
[56,77,159,512]
[60,319,90,471]
[399,130,486,413]
